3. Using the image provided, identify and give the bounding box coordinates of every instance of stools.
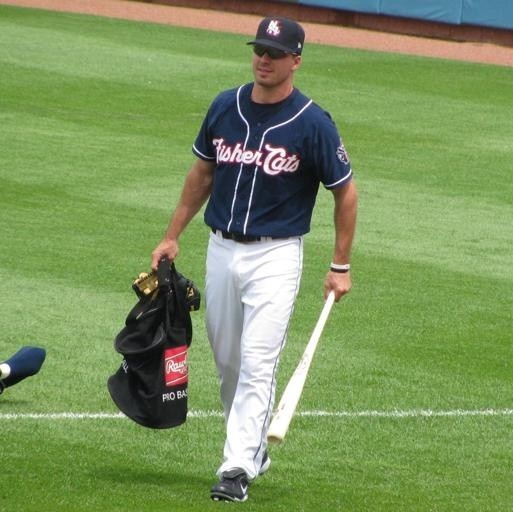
[211,226,281,242]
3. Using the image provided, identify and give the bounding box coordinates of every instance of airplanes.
[133,268,201,310]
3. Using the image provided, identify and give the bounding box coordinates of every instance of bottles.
[246,17,305,54]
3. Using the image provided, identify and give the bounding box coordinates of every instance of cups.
[253,45,287,58]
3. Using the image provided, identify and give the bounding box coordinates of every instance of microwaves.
[268,288,336,444]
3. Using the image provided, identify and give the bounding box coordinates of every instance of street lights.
[106,256,200,429]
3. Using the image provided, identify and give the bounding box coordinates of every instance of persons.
[151,17,356,503]
[0,346,46,396]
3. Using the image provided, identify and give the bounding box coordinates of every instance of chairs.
[210,451,271,502]
